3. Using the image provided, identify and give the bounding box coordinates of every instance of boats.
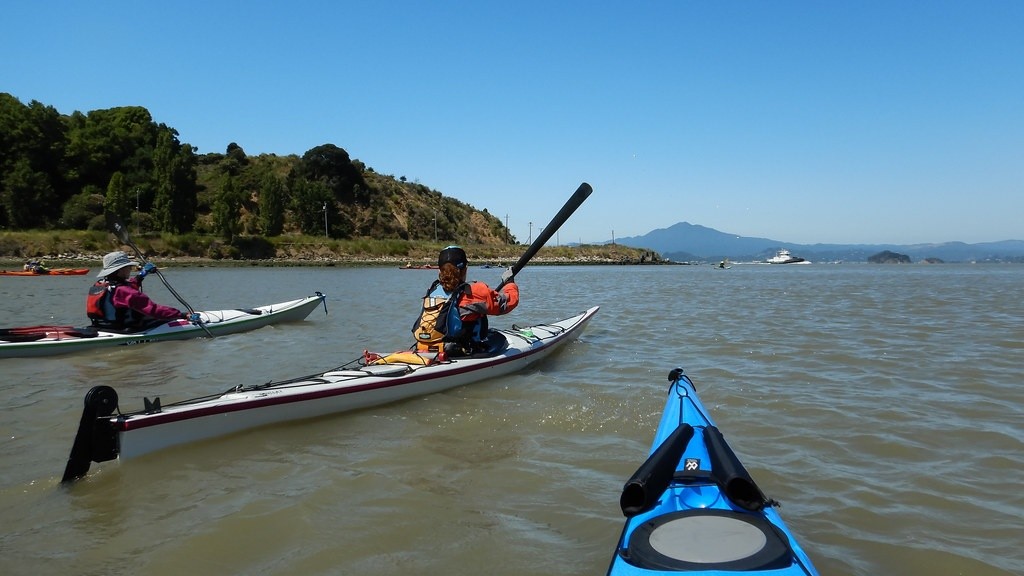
[0,268,89,276]
[0,291,325,359]
[766,248,812,264]
[107,305,600,463]
[714,266,732,270]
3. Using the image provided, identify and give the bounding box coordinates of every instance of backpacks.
[411,282,472,343]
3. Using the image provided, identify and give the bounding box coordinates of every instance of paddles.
[494,182,595,294]
[114,222,216,340]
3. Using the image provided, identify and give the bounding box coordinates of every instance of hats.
[438,246,469,270]
[96,251,140,278]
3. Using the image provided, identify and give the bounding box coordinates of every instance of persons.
[86,251,192,333]
[412,248,519,355]
[22,258,48,274]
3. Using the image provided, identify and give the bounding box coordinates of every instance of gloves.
[188,313,201,321]
[139,262,157,277]
[501,266,514,283]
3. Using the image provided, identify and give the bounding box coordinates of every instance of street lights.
[135,187,140,234]
[323,202,328,238]
[530,222,533,245]
